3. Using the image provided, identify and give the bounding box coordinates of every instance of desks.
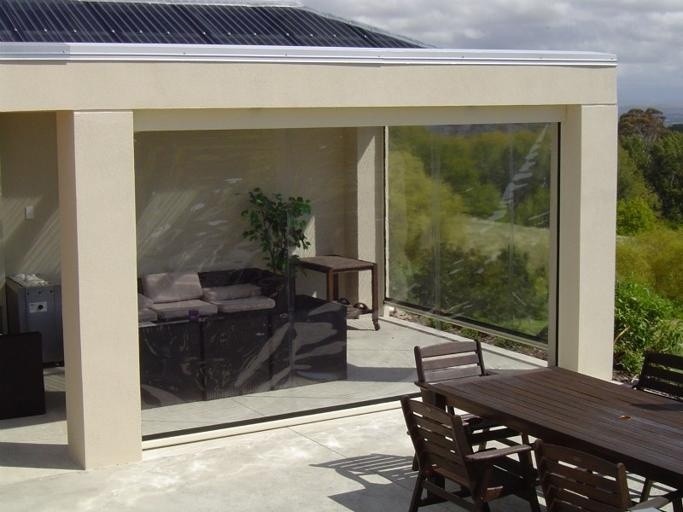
[289,255,380,331]
[425,366,682,501]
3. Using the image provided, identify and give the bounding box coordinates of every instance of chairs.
[632,350,683,503]
[533,438,683,512]
[413,336,528,452]
[401,395,543,512]
[1,332,45,420]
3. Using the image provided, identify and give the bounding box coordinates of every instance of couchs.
[136,266,348,410]
